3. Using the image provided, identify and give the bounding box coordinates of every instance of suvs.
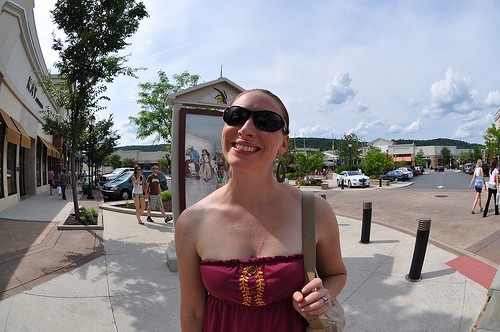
[99,167,135,186]
[100,170,169,201]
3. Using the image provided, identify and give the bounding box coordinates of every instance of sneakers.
[165,217,173,223]
[147,216,154,222]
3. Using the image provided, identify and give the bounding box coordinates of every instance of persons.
[174,86,348,332]
[146,164,173,223]
[131,165,147,224]
[49,167,89,200]
[358,167,362,173]
[468,158,487,214]
[481,159,500,217]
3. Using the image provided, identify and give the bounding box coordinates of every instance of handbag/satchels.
[57,185,62,195]
[496,193,500,205]
[302,190,346,332]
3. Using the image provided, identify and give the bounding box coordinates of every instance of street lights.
[86,115,96,199]
[348,142,352,170]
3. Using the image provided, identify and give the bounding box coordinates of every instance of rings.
[322,296,328,305]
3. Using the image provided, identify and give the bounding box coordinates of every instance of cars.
[463,163,490,176]
[336,170,371,188]
[379,166,424,183]
[435,165,445,172]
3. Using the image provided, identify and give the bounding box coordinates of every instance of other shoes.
[495,212,500,215]
[471,210,475,214]
[480,207,483,211]
[139,220,144,224]
[483,215,487,217]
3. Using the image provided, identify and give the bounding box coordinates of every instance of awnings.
[0,109,62,159]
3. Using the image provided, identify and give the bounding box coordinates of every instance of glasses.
[223,106,287,133]
[138,169,141,170]
